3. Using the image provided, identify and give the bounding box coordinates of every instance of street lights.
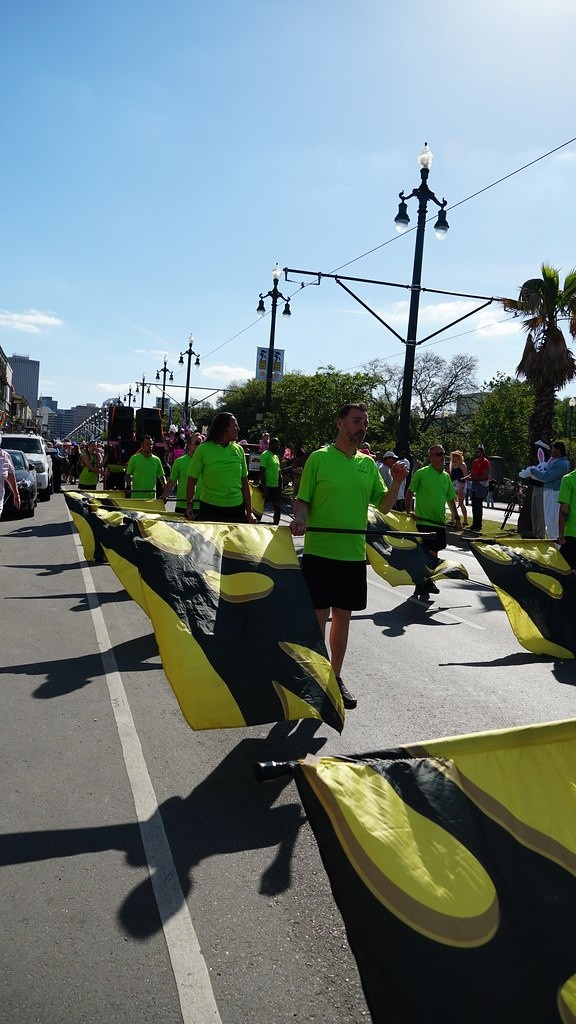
[124,385,136,408]
[255,262,292,420]
[135,373,151,410]
[391,142,451,510]
[65,394,123,443]
[178,333,201,427]
[155,352,174,421]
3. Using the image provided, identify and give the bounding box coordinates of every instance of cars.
[0,449,38,519]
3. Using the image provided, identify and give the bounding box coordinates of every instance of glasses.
[387,457,395,460]
[432,452,445,456]
[189,441,201,446]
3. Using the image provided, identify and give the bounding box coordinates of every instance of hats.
[383,451,399,458]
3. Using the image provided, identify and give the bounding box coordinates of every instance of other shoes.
[414,585,430,599]
[462,523,469,526]
[446,522,455,525]
[424,578,440,594]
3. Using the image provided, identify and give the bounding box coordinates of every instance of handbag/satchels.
[72,450,90,477]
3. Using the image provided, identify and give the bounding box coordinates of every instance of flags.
[468,540,576,660]
[365,504,469,587]
[63,491,345,736]
[291,736,576,1024]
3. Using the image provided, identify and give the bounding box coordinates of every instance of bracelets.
[455,516,461,520]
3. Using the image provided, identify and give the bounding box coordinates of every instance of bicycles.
[500,477,529,531]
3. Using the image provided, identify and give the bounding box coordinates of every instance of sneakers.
[337,676,357,706]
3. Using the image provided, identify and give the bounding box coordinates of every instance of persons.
[556,468,576,548]
[466,448,491,528]
[530,442,570,539]
[359,442,410,511]
[186,412,256,523]
[126,435,166,499]
[405,444,463,557]
[486,480,496,507]
[290,402,409,705]
[258,433,270,452]
[257,437,283,525]
[0,433,21,516]
[47,441,108,493]
[159,433,203,514]
[446,449,469,526]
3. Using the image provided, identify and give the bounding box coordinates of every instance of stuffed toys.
[519,449,555,480]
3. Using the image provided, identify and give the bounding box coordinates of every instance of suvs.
[0,430,57,502]
